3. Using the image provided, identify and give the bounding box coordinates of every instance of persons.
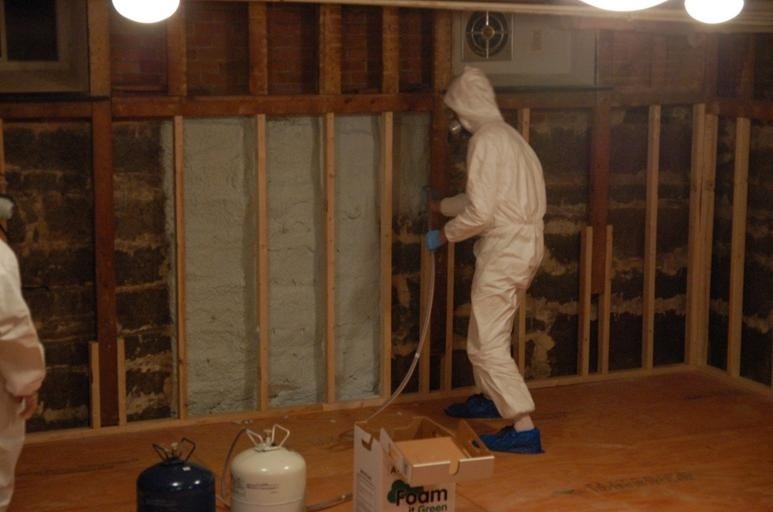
[423,65,547,456]
[0,171,48,511]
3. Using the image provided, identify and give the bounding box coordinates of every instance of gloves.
[425,230,442,250]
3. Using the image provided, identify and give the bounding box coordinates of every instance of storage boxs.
[354,415,495,512]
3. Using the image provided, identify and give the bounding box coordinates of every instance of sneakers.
[443,391,501,419]
[472,425,541,455]
